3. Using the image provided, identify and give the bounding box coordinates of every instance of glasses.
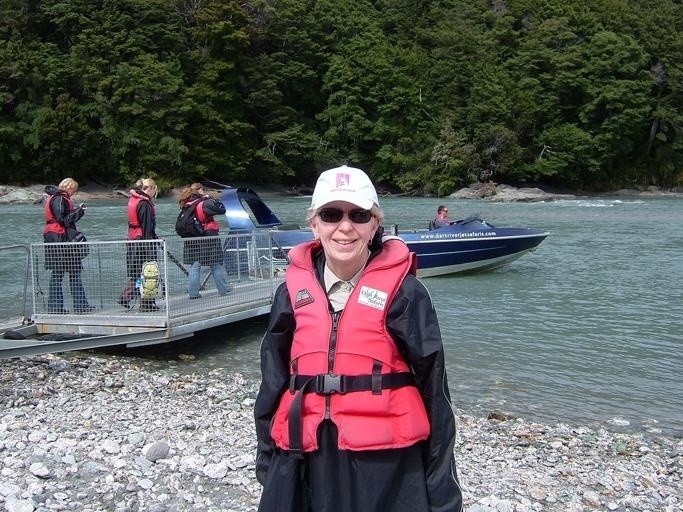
[318,208,372,224]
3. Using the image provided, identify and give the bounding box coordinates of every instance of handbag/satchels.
[61,217,90,265]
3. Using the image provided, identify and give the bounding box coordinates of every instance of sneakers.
[116,297,130,310]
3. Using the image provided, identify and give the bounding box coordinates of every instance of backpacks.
[139,261,164,298]
[176,199,205,237]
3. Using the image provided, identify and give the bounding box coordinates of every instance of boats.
[216,188,550,279]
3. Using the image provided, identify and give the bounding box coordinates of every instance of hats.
[311,164,379,210]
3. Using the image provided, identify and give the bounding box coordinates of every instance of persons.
[41,178,97,315]
[427,205,461,228]
[116,177,161,314]
[253,166,460,511]
[177,182,234,300]
